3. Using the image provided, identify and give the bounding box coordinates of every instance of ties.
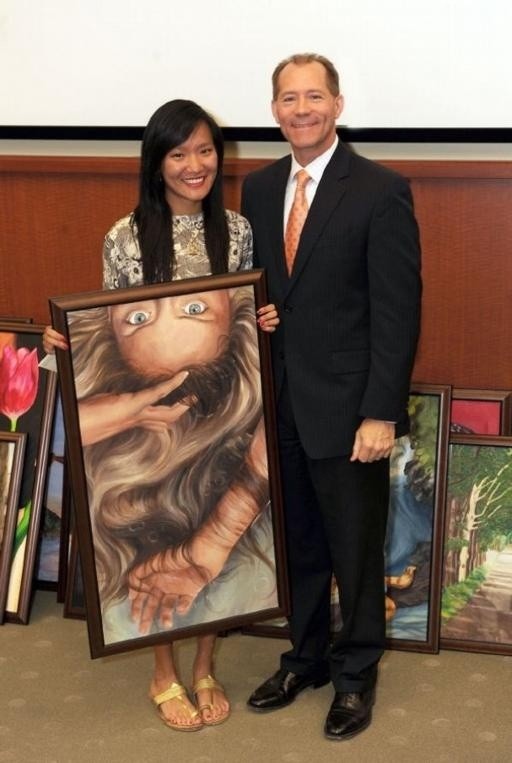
[285,170,311,279]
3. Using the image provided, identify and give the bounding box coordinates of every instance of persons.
[65,281,272,643]
[247,50,424,742]
[39,97,282,730]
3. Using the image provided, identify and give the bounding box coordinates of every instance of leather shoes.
[247,663,331,714]
[324,676,376,741]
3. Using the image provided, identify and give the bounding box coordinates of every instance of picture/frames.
[48,267,291,662]
[0,321,65,630]
[56,383,512,656]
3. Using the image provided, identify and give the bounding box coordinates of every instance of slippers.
[190,672,231,728]
[148,683,203,733]
